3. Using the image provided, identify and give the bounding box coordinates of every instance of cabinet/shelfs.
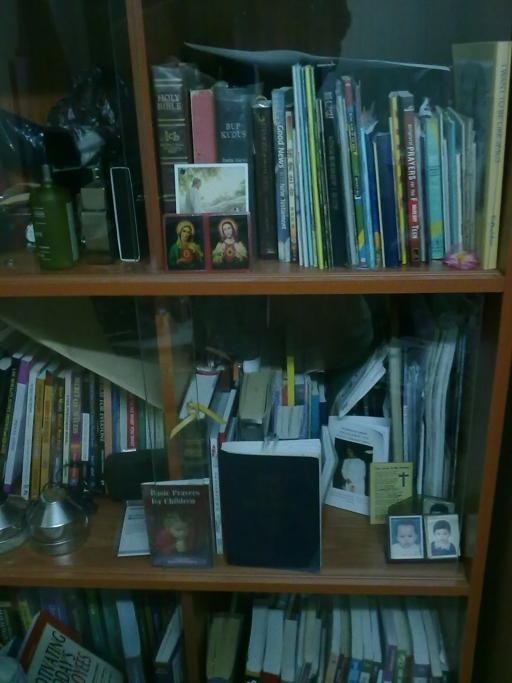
[0,0,512,683]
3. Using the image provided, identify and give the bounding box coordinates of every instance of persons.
[158,511,196,553]
[430,520,457,555]
[429,503,449,515]
[392,520,420,555]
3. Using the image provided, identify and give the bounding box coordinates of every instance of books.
[218,436,326,573]
[150,36,510,273]
[0,314,471,525]
[141,478,215,570]
[0,590,452,682]
